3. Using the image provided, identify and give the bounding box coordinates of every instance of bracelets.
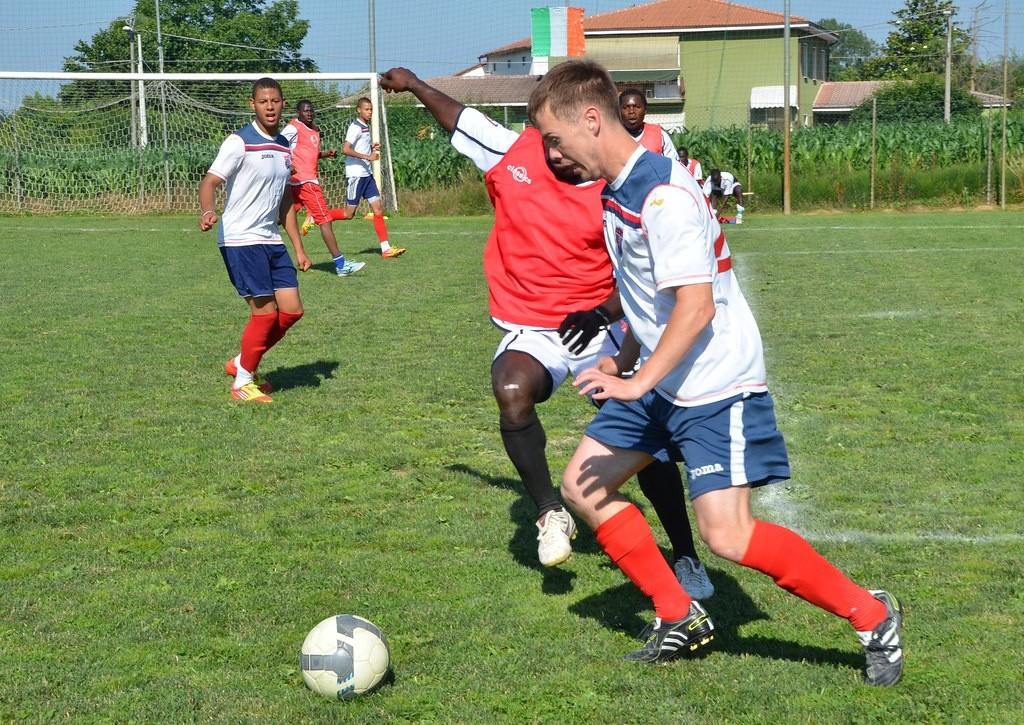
[203,210,217,215]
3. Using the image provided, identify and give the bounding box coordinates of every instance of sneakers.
[627,601,717,662]
[301,210,316,236]
[735,213,742,224]
[336,261,366,277]
[536,503,579,566]
[673,556,713,600]
[231,381,272,404]
[857,591,902,688]
[381,245,407,259]
[225,357,272,392]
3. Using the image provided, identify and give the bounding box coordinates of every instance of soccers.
[300,614,390,701]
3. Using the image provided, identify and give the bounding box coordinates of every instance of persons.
[619,88,681,164]
[527,58,905,685]
[677,147,705,186]
[302,97,406,258]
[703,169,744,224]
[199,78,312,403]
[378,67,715,601]
[279,101,366,277]
